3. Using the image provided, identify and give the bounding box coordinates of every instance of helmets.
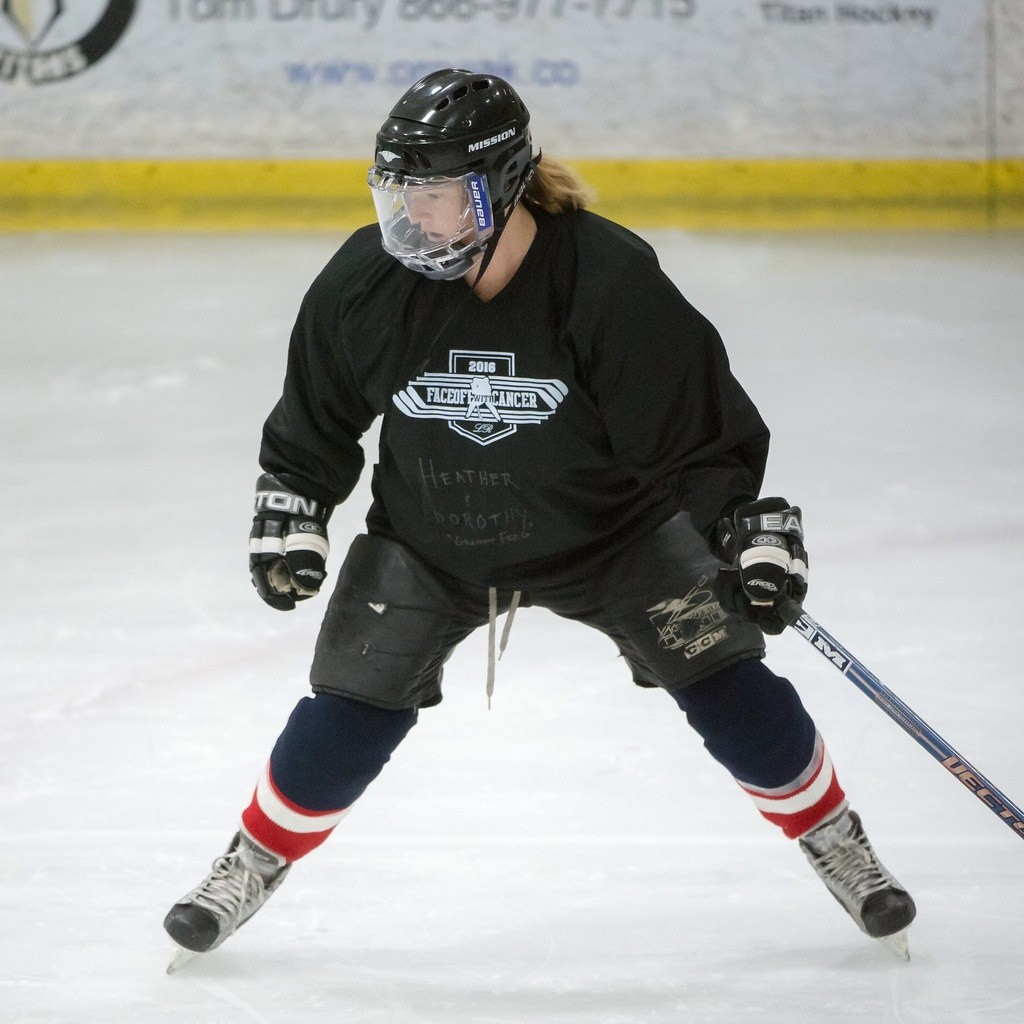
[371,68,534,282]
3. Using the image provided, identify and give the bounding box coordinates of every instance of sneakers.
[162,831,297,952]
[797,812,916,939]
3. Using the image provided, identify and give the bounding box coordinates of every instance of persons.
[162,66,923,953]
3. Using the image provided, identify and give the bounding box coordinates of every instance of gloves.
[250,474,340,612]
[721,497,808,633]
